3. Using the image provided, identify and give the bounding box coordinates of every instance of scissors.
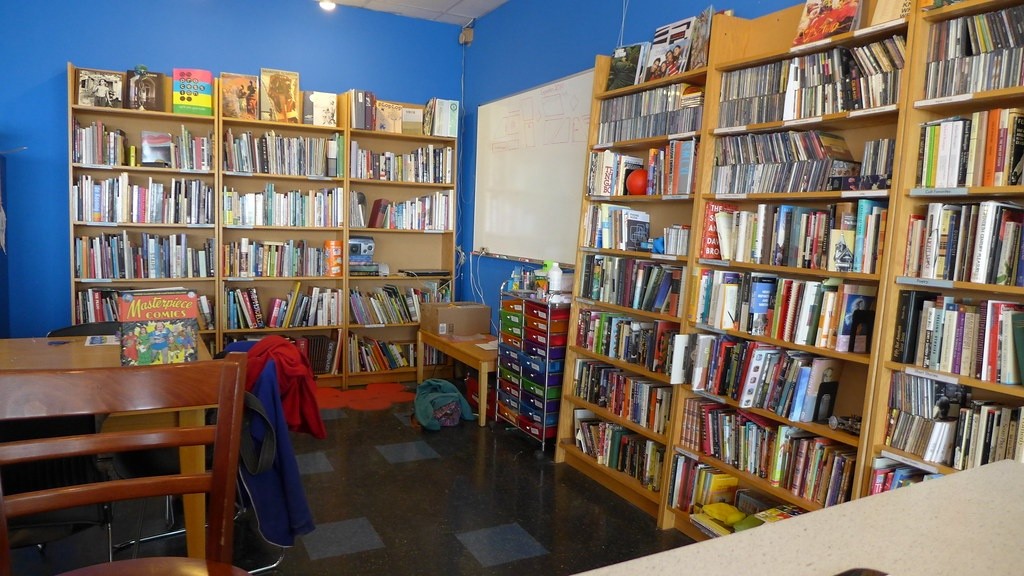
[47,340,77,346]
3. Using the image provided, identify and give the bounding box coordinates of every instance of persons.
[90,79,112,107]
[645,45,688,81]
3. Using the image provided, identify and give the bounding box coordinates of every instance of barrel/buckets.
[323,240,343,277]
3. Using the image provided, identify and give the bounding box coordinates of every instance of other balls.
[625,169,648,195]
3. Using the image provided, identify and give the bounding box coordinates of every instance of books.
[640,16,695,82]
[720,0,1024,129]
[574,106,1024,538]
[346,89,459,373]
[598,78,705,144]
[220,69,344,375]
[686,5,713,72]
[605,43,652,93]
[72,117,217,366]
[75,68,213,117]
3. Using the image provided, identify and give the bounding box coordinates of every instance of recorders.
[348,235,375,255]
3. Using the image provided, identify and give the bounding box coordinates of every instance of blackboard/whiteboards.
[471,67,594,270]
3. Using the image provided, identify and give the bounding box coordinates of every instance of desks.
[411,333,502,427]
[0,321,219,560]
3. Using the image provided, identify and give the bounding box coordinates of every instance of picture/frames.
[76,66,127,109]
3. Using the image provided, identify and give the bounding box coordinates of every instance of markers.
[491,254,508,259]
[472,252,478,255]
[521,265,534,290]
[518,258,530,262]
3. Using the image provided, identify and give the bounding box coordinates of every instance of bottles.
[507,259,575,303]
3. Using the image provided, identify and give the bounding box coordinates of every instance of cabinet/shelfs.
[557,0,1024,539]
[67,61,467,398]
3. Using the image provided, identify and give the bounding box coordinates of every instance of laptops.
[398,268,450,277]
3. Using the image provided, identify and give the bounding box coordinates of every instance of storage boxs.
[420,301,492,336]
[496,276,570,440]
[733,486,778,515]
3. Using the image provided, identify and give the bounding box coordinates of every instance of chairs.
[48,320,174,533]
[0,359,250,576]
[113,334,330,574]
